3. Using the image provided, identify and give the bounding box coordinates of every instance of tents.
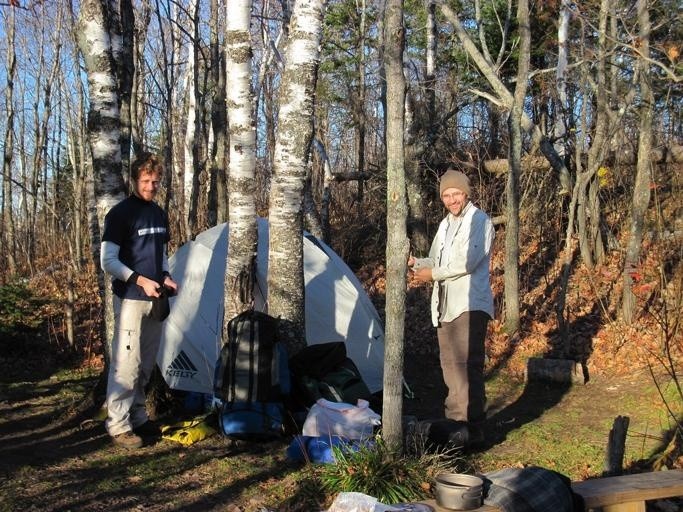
[152,216,418,411]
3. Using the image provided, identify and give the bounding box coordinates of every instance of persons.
[96,150,182,451]
[405,167,499,427]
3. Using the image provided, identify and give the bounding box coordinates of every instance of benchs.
[419,466,682,512]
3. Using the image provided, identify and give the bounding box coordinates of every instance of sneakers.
[134,421,163,435]
[426,421,494,454]
[112,431,144,449]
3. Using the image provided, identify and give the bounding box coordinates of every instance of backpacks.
[288,341,375,410]
[216,398,284,442]
[212,312,292,402]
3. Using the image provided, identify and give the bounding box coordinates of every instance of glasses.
[441,191,462,199]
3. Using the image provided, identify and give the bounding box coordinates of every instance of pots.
[434,471,484,509]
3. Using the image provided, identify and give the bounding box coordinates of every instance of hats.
[439,168,471,199]
